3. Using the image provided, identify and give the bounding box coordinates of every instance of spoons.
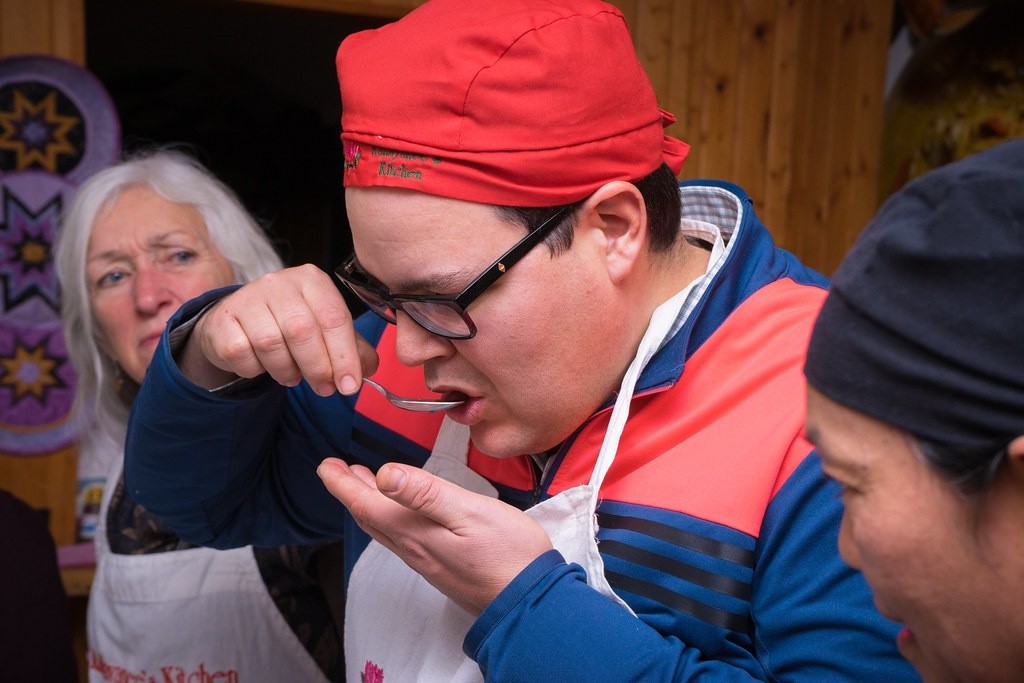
[364,375,466,413]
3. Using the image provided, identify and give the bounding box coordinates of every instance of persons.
[106,3,916,682]
[803,143,1024,683]
[52,146,347,683]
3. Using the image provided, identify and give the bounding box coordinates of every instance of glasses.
[333,205,579,340]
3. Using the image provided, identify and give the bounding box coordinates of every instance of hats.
[336,0,693,207]
[801,136,1024,449]
